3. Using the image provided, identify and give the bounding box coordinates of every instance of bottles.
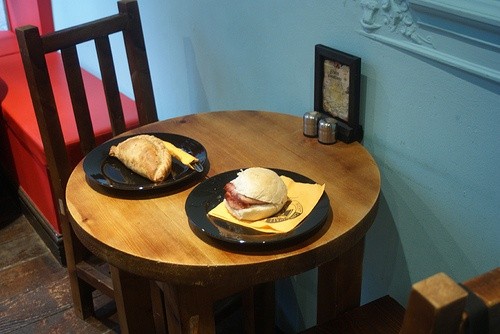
[318,118,338,143]
[303,111,321,137]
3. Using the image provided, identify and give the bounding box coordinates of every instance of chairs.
[295,266,500,334]
[14,0,254,334]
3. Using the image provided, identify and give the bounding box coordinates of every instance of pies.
[108,135,172,182]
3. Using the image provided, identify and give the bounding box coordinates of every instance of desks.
[65,110,381,334]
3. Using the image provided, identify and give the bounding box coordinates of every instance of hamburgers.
[223,167,287,221]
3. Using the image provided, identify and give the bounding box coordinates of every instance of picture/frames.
[314,45,361,130]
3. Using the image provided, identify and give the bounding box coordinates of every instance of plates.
[83,132,207,190]
[186,166,330,245]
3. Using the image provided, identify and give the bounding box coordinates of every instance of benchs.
[0,50,142,267]
